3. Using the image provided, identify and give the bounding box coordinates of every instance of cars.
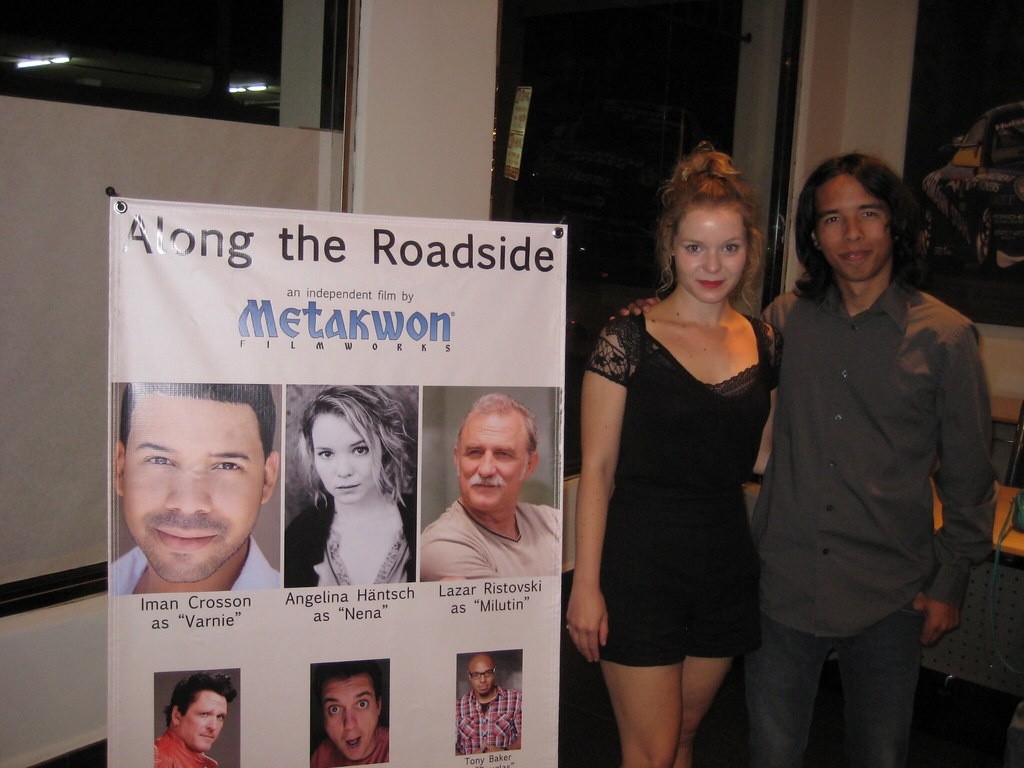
[918,98,1024,275]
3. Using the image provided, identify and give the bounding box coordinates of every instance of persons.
[419,391,560,580]
[455,653,521,755]
[108,382,281,596]
[154,671,236,768]
[310,660,389,767]
[565,140,776,768]
[285,385,415,588]
[619,152,995,768]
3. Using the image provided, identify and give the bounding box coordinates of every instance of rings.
[565,624,576,629]
